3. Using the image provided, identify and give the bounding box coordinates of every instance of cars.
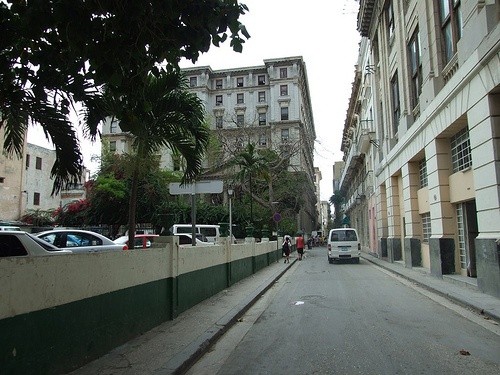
[0,224,220,256]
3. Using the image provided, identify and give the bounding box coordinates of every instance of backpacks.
[283,242,289,251]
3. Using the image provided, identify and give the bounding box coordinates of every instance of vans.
[327,227,362,264]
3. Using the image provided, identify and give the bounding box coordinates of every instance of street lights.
[272,201,280,262]
[227,187,235,242]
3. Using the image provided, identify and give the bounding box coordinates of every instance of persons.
[307,235,326,249]
[296,233,305,260]
[284,232,291,245]
[282,237,292,264]
